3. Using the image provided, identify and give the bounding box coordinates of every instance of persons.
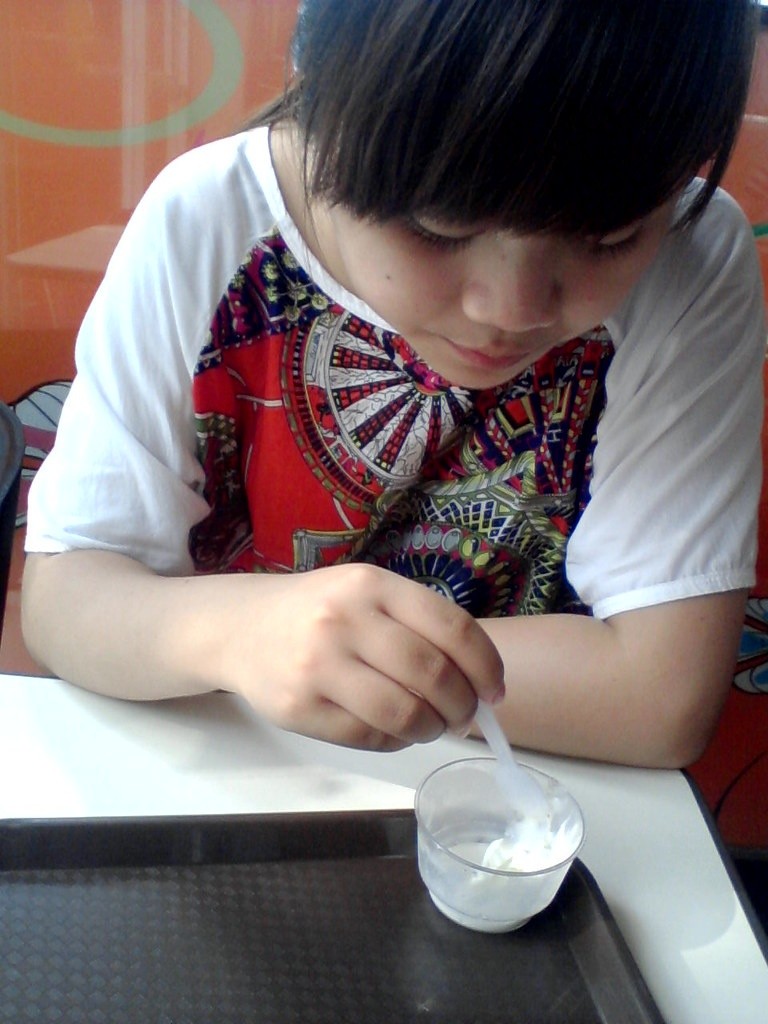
[16,0,768,768]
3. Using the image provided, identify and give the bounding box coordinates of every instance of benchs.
[0,0,768,859]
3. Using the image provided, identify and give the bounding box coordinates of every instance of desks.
[0,675,768,1024]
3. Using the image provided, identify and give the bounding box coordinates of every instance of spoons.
[476,700,545,814]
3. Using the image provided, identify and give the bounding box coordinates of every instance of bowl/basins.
[413,757,586,934]
